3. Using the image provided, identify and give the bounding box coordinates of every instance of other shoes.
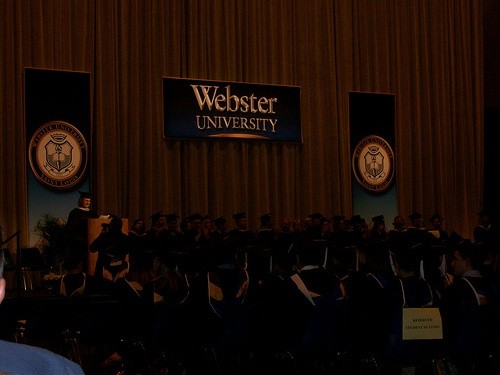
[80,272,89,282]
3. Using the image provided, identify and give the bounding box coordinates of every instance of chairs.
[14,295,500,375]
[2,248,40,291]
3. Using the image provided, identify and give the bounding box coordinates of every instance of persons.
[0,234,85,375]
[0,190,500,375]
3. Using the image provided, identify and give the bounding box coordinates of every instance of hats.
[185,213,205,223]
[320,216,331,224]
[213,215,226,226]
[293,219,302,225]
[373,215,384,224]
[257,213,272,222]
[429,215,443,222]
[350,215,362,226]
[408,212,421,220]
[309,212,323,221]
[477,211,492,217]
[281,217,290,224]
[182,217,192,226]
[3,245,482,303]
[332,216,345,221]
[151,211,166,221]
[78,191,97,202]
[304,218,312,224]
[164,215,180,222]
[232,212,247,221]
